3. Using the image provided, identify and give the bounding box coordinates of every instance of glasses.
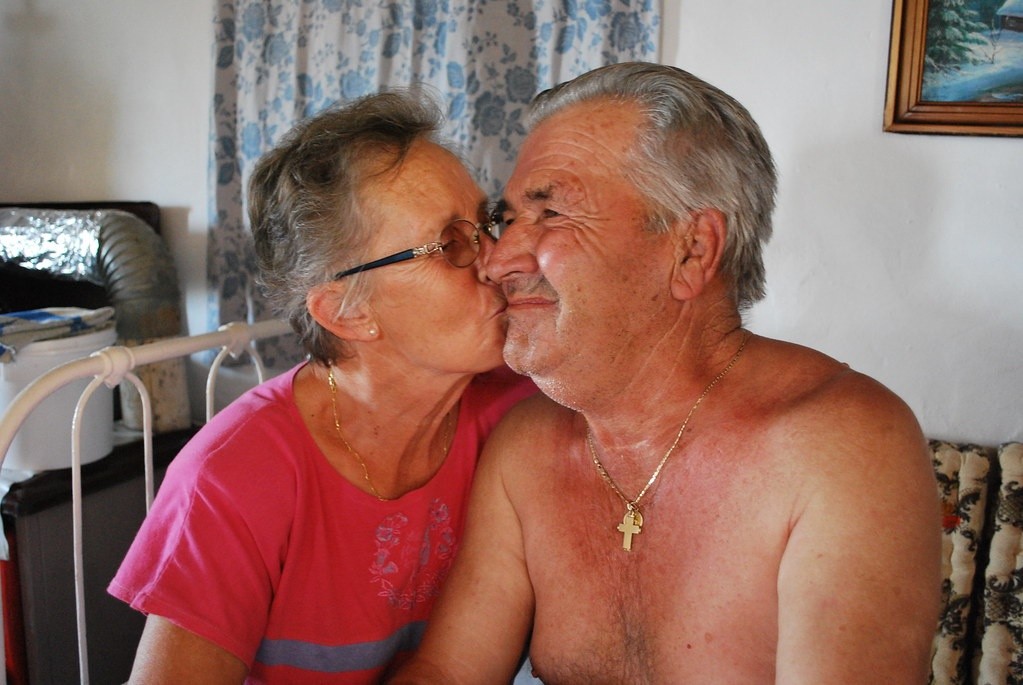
[333,208,500,281]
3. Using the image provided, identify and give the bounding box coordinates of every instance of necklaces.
[589,335,747,552]
[328,371,451,500]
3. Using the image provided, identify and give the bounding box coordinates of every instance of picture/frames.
[882,0,1023,139]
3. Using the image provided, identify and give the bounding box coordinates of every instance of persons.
[388,61,940,685]
[107,89,542,685]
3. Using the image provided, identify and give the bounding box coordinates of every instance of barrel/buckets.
[1,321,113,472]
